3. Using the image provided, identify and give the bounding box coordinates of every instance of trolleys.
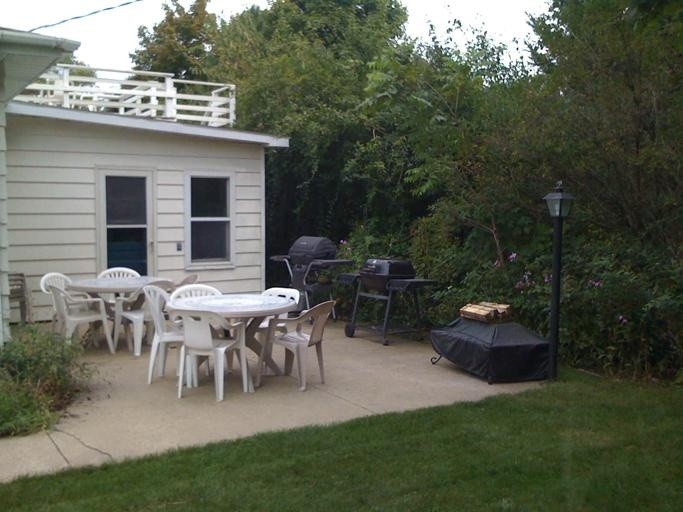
[339,254,441,346]
[268,234,354,325]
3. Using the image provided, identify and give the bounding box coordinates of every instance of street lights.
[541,178,577,381]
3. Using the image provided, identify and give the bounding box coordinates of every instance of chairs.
[7,271,35,325]
[37,267,198,357]
[142,283,335,403]
